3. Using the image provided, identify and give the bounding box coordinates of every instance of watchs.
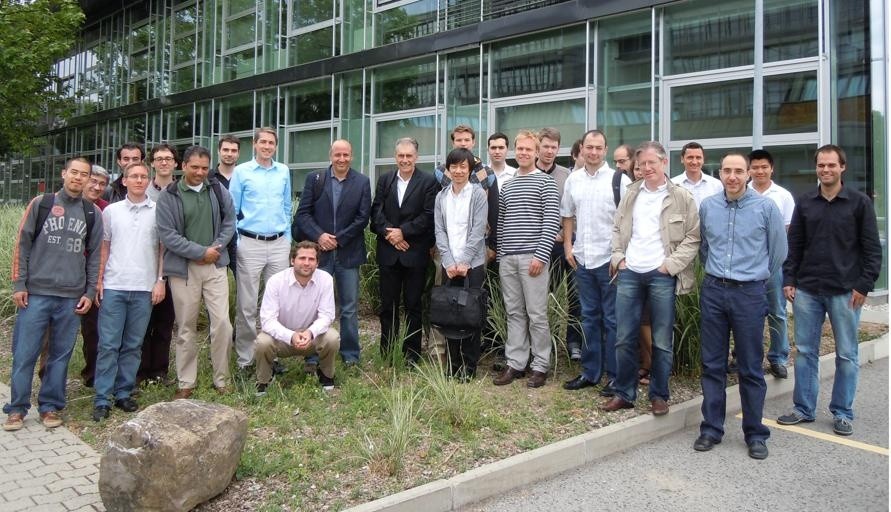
[156,275,168,282]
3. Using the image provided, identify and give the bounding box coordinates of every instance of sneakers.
[39,409,62,427]
[317,369,334,391]
[4,411,25,431]
[834,413,854,434]
[255,372,276,397]
[778,409,815,424]
[728,356,738,372]
[771,364,790,379]
[568,344,583,360]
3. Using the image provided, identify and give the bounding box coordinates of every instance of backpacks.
[35,192,95,250]
[370,169,398,234]
[291,169,325,243]
[612,169,629,208]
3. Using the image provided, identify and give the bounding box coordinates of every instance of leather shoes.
[115,397,139,411]
[652,396,669,415]
[599,379,617,396]
[218,385,234,394]
[694,433,722,450]
[493,364,526,385]
[749,439,768,459]
[172,388,193,401]
[94,405,110,421]
[601,396,634,411]
[563,374,594,390]
[528,370,548,387]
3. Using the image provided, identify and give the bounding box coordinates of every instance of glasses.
[612,157,631,167]
[153,157,175,163]
[720,169,751,177]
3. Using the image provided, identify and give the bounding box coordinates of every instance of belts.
[237,229,284,241]
[713,275,743,284]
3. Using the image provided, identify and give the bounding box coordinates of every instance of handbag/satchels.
[427,273,486,338]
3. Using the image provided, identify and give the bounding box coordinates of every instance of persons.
[775,143,883,436]
[38,165,111,388]
[629,148,652,386]
[560,130,634,397]
[487,133,518,194]
[295,140,372,376]
[253,241,341,397]
[207,134,242,343]
[434,124,505,371]
[228,129,292,384]
[669,142,725,214]
[434,147,488,383]
[136,144,177,388]
[613,145,635,183]
[80,144,147,388]
[493,128,560,387]
[598,141,701,416]
[94,163,165,422]
[694,152,788,460]
[3,157,103,431]
[370,138,443,370]
[535,126,581,361]
[155,145,233,403]
[727,150,796,379]
[568,140,584,173]
